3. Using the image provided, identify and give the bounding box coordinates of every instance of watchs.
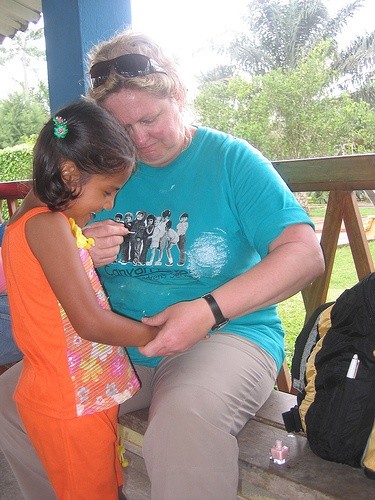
[201,293,230,330]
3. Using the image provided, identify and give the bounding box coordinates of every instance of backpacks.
[281,272,375,479]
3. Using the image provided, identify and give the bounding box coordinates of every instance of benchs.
[0,151,375,500]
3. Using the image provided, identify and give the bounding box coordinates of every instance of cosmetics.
[266,438,291,465]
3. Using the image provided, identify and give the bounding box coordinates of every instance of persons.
[0,99,164,499]
[0,35,326,499]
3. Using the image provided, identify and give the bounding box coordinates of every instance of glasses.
[90,54,167,89]
